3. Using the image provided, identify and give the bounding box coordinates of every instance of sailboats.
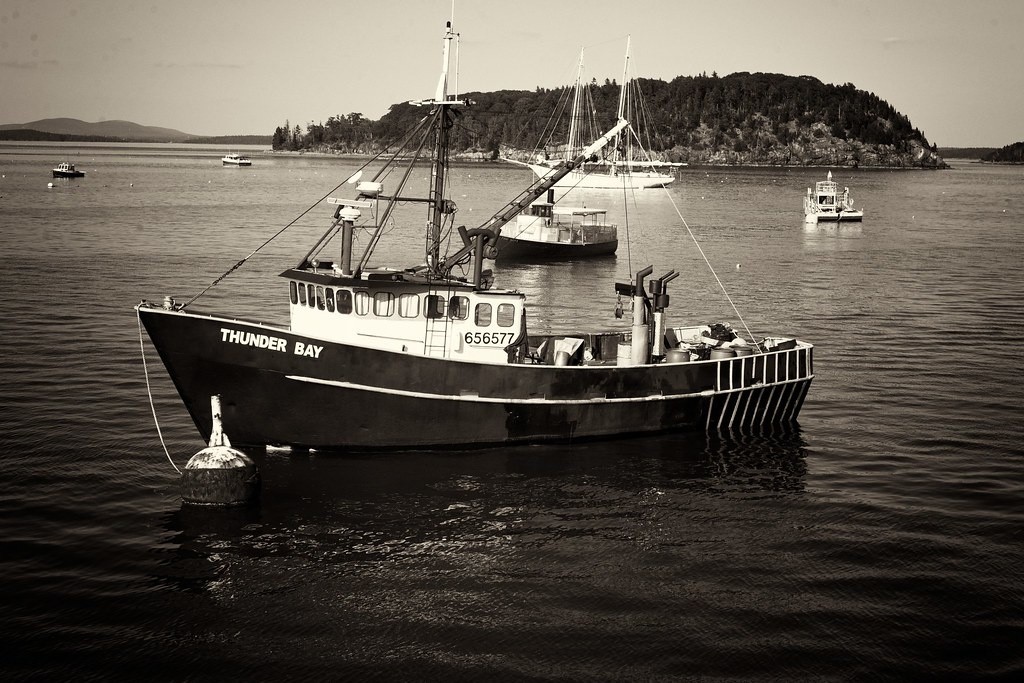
[525,46,689,168]
[506,33,676,189]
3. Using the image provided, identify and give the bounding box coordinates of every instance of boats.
[132,1,815,453]
[221,149,252,166]
[52,161,85,179]
[469,173,619,259]
[803,170,865,222]
